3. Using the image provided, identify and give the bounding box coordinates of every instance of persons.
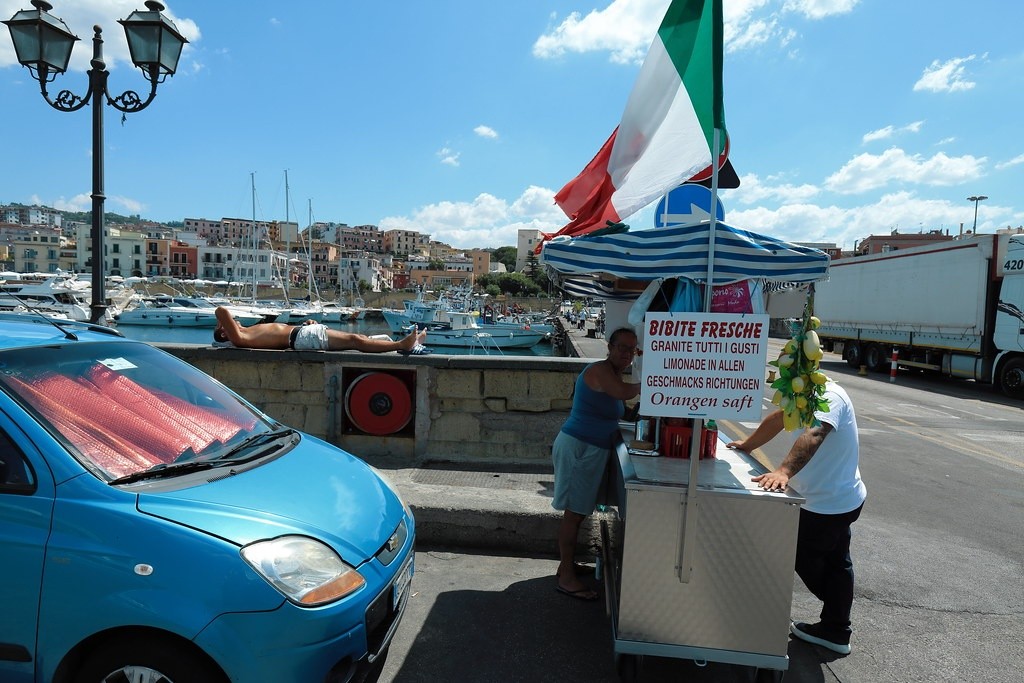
[503,301,522,317]
[214,306,427,352]
[726,350,867,654]
[552,327,642,602]
[566,309,587,331]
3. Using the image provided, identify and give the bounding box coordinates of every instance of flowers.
[767,282,838,432]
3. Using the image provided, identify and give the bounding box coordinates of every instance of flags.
[534,0,726,256]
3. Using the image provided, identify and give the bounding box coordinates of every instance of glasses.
[613,343,640,355]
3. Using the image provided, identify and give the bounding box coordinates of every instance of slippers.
[396,341,434,354]
[556,585,606,600]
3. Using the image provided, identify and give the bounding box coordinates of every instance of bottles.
[634,416,650,442]
[705,420,718,459]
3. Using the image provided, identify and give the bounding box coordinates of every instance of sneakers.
[790,620,852,655]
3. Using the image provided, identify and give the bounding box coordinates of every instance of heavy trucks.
[814,234,1024,398]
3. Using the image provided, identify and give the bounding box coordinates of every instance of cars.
[599,310,606,318]
[0,311,416,683]
[587,312,598,319]
[563,300,572,306]
[578,310,586,317]
[581,301,588,306]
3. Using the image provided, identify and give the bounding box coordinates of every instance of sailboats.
[111,169,383,327]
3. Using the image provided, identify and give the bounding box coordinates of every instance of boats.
[0,271,93,319]
[383,290,556,349]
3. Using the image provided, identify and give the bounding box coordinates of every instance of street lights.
[967,196,988,234]
[854,240,858,256]
[0,0,191,327]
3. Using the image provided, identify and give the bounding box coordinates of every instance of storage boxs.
[661,419,707,460]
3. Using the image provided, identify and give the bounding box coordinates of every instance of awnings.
[540,220,832,305]
